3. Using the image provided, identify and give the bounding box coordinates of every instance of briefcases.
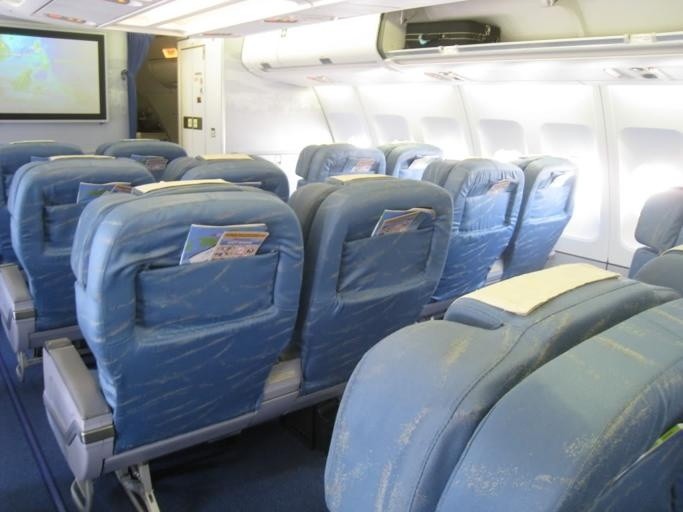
[405,20,501,47]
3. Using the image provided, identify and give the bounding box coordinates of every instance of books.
[545,170,570,189]
[342,157,375,174]
[76,182,134,202]
[375,212,423,237]
[112,184,132,196]
[491,179,510,196]
[350,160,372,174]
[181,222,270,263]
[371,208,436,233]
[208,231,268,259]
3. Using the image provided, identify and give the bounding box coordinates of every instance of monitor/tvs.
[0,25,107,122]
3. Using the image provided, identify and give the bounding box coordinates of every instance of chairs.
[2,137,682,510]
